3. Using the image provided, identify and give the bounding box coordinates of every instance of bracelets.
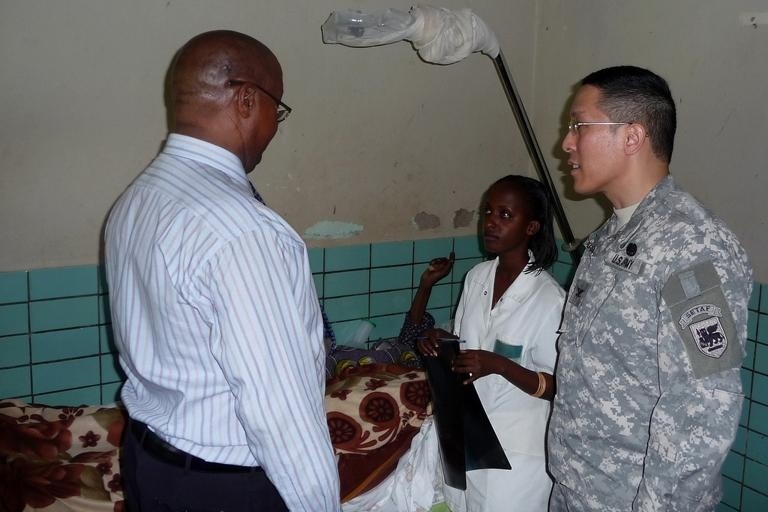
[532,372,547,398]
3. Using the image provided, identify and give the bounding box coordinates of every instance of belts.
[128,418,265,476]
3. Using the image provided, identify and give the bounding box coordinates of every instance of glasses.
[227,80,293,123]
[567,119,629,132]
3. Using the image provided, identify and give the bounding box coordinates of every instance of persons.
[323,251,456,383]
[416,175,570,511]
[96,27,346,512]
[543,65,754,511]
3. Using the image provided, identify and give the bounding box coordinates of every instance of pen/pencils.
[413,336,466,343]
